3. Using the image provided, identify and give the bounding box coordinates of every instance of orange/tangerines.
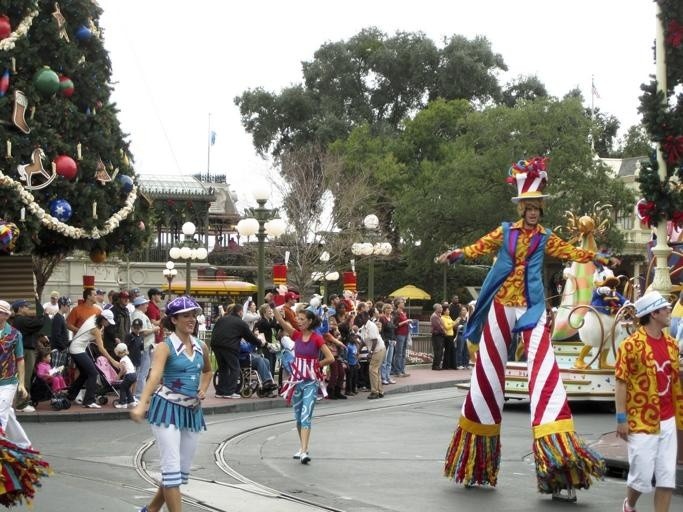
[29,358,72,410]
[86,340,124,407]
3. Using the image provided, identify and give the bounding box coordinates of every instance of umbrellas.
[388,284,431,321]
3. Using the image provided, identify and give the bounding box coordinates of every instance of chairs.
[382,372,410,385]
[215,392,241,398]
[294,450,310,464]
[17,405,36,412]
[623,498,636,512]
[115,402,136,409]
[326,386,384,399]
[82,402,102,408]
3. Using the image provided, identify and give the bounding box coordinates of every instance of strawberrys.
[212,352,269,399]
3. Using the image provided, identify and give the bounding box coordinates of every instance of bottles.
[0,300,30,314]
[165,296,202,316]
[133,319,142,326]
[101,309,116,325]
[635,290,671,317]
[133,297,150,305]
[51,291,71,305]
[114,343,129,357]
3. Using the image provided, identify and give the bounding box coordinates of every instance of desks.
[352,214,392,305]
[238,182,286,310]
[311,250,338,305]
[162,261,178,293]
[169,222,207,297]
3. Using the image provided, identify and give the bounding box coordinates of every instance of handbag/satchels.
[614,412,627,423]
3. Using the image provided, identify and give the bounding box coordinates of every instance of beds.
[388,284,431,321]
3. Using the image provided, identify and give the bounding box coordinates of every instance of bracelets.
[614,412,627,423]
[370,350,374,354]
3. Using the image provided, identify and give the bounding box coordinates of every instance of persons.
[103,289,166,408]
[130,296,214,512]
[266,296,334,466]
[211,290,348,401]
[0,298,32,453]
[14,289,124,409]
[432,199,621,503]
[197,307,207,331]
[428,294,471,371]
[612,289,682,511]
[337,290,412,399]
